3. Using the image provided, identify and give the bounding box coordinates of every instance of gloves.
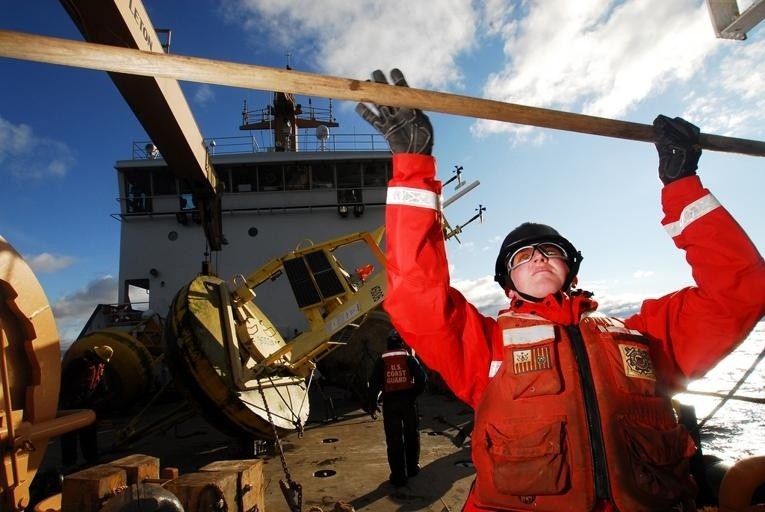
[355,68,433,155]
[653,114,702,185]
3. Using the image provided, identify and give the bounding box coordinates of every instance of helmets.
[495,222,584,290]
[387,328,405,349]
[93,345,113,363]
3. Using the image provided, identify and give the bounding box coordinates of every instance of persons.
[355,68,765,512]
[58,346,114,466]
[283,118,292,152]
[362,329,428,487]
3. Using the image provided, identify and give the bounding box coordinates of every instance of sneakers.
[389,463,420,486]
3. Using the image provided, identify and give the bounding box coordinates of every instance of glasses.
[507,242,569,276]
